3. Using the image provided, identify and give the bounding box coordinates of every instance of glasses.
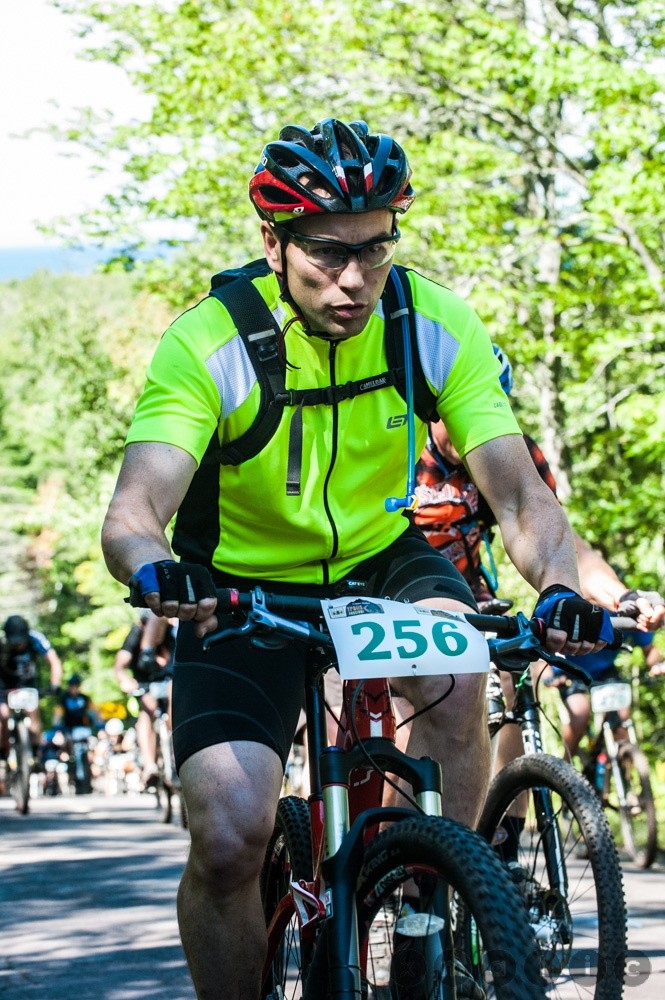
[274,210,402,272]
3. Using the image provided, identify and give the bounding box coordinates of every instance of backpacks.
[169,255,440,574]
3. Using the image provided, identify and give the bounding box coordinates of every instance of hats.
[67,672,84,686]
[2,615,30,645]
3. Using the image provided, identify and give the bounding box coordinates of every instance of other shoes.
[505,854,532,885]
[141,771,159,786]
[626,789,642,818]
[455,956,488,999]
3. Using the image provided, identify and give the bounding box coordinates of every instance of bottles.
[596,753,607,789]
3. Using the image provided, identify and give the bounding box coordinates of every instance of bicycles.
[118,578,601,1000]
[1,667,660,871]
[442,610,660,1000]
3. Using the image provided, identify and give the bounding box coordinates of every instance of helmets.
[138,607,152,624]
[491,341,515,397]
[247,116,420,231]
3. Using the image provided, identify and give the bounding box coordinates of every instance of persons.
[100,119,615,1000]
[536,543,665,820]
[0,615,62,792]
[36,671,142,796]
[116,606,178,787]
[297,342,665,913]
